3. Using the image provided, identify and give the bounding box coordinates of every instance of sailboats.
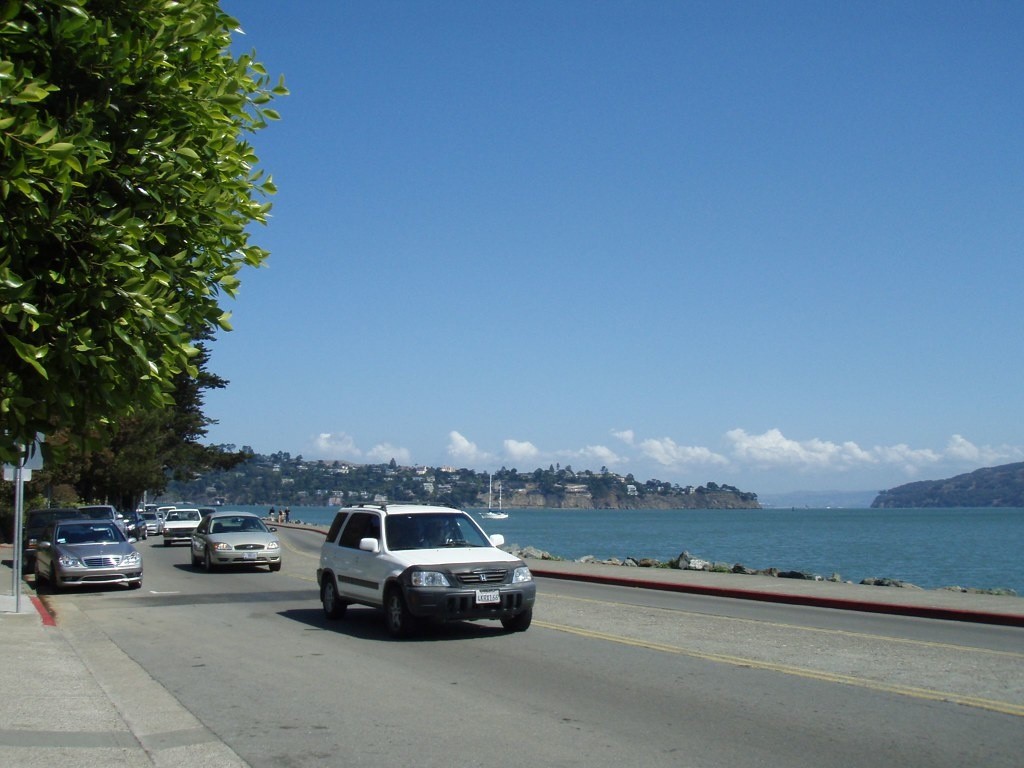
[478,471,509,519]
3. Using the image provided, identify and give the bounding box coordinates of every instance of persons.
[269,506,290,523]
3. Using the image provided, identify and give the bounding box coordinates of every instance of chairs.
[213,522,225,533]
[240,519,255,529]
[188,513,195,518]
[171,516,179,519]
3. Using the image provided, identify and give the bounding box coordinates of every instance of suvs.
[162,509,203,547]
[78,505,129,542]
[24,507,87,574]
[316,503,537,638]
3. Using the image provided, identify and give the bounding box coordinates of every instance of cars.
[198,507,217,521]
[190,510,282,574]
[119,512,147,542]
[136,502,177,536]
[33,518,143,594]
